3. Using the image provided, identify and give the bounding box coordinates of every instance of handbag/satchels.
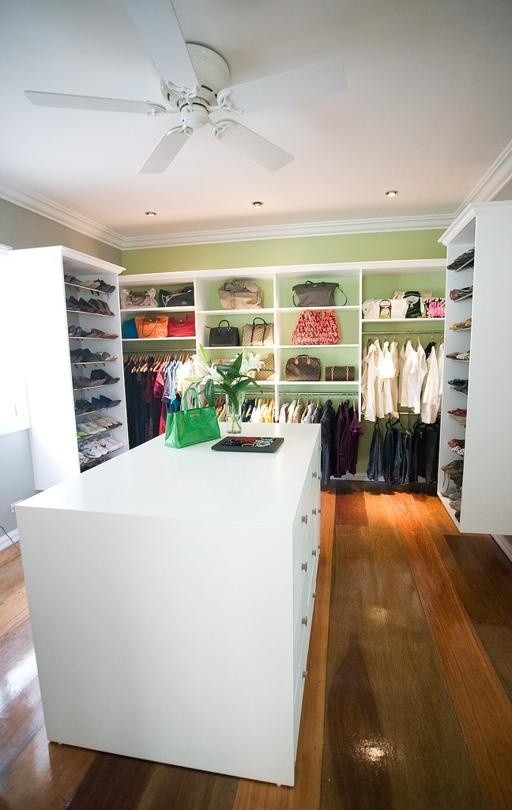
[135,316,169,338]
[168,316,195,336]
[363,298,407,319]
[120,288,161,307]
[218,280,265,310]
[326,366,355,380]
[292,280,348,306]
[166,388,222,449]
[242,317,274,346]
[123,319,140,338]
[423,297,446,318]
[286,355,321,381]
[209,320,241,347]
[159,286,195,306]
[393,289,433,319]
[288,310,343,345]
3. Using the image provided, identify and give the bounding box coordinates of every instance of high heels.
[67,295,115,316]
[73,369,121,388]
[75,394,122,414]
[76,278,94,292]
[71,348,119,369]
[98,280,116,295]
[91,279,111,294]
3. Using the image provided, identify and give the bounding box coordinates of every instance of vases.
[222,414,244,435]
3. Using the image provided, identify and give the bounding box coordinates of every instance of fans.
[22,1,346,178]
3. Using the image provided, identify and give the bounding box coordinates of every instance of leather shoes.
[446,248,474,270]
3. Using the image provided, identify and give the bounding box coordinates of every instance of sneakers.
[86,422,106,432]
[446,407,468,428]
[446,352,460,358]
[98,439,119,453]
[80,442,101,458]
[450,474,463,480]
[97,414,119,424]
[77,431,88,438]
[441,486,464,524]
[455,478,463,486]
[450,286,472,301]
[79,451,89,463]
[78,422,99,434]
[440,460,464,472]
[448,378,470,395]
[105,437,123,447]
[69,323,119,341]
[446,464,463,473]
[449,317,471,331]
[448,438,466,456]
[77,435,81,438]
[91,415,112,427]
[465,354,470,361]
[456,352,469,360]
[85,439,109,454]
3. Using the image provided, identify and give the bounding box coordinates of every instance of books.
[209,436,284,454]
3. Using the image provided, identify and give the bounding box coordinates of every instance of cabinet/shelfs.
[120,264,445,492]
[436,199,512,538]
[11,422,323,747]
[8,244,124,493]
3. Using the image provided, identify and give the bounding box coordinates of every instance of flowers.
[182,341,271,408]
